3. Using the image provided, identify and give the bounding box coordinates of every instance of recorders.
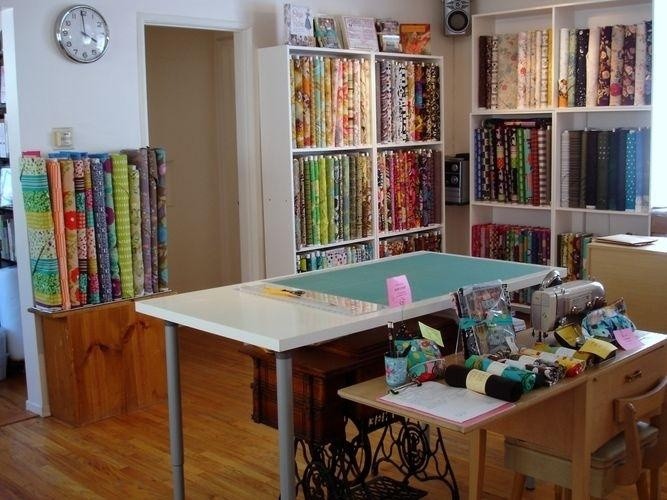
[530,281,605,331]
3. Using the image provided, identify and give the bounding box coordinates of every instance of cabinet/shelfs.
[468,2,654,316]
[28,284,178,425]
[257,40,445,280]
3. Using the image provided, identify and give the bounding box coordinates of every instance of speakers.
[445,153,469,205]
[442,0,471,37]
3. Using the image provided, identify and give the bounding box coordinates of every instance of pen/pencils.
[387,321,411,357]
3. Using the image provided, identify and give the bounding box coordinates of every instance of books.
[282,4,431,55]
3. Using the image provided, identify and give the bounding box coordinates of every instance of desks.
[134,246,568,500]
[335,324,666,500]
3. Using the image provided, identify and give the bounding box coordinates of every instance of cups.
[383,354,406,387]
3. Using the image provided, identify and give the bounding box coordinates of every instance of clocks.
[51,1,112,64]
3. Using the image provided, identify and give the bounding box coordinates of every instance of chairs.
[501,378,667,500]
